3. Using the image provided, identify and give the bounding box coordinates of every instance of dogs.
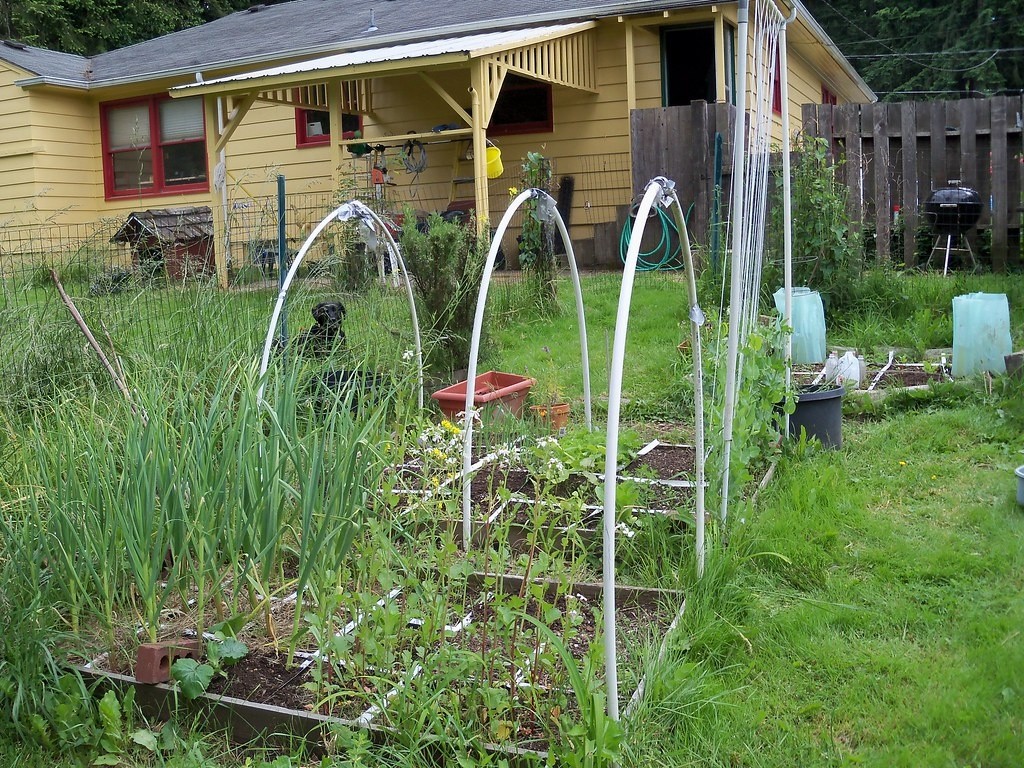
[271,300,347,360]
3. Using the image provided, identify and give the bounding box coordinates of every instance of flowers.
[525,345,566,406]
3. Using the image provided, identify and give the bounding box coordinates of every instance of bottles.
[837,351,859,389]
[832,351,838,362]
[857,355,867,384]
[826,354,837,381]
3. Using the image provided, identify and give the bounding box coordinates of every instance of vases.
[431,370,537,424]
[530,403,569,429]
[312,370,386,421]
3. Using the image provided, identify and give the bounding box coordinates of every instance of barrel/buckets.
[772,384,846,452]
[486,147,504,179]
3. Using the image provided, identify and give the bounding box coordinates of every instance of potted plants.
[771,359,852,450]
[399,202,490,394]
